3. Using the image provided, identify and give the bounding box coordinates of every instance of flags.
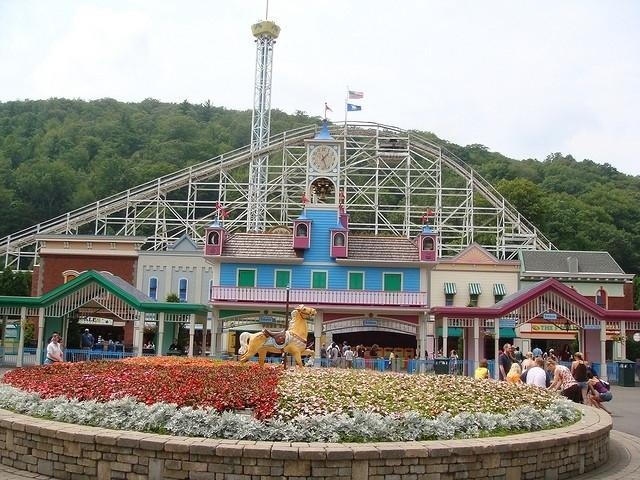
[302,194,310,203]
[349,91,363,99]
[419,209,433,223]
[347,104,361,111]
[326,105,333,112]
[216,199,230,218]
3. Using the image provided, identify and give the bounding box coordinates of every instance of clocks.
[306,140,341,175]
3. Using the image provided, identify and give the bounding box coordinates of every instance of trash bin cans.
[433,355,451,374]
[591,362,601,377]
[616,358,636,387]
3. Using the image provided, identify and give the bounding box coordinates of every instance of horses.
[236,304,318,367]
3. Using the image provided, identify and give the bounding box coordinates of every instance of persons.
[416,345,458,373]
[169,338,182,353]
[43,331,65,364]
[80,328,117,353]
[475,342,614,415]
[143,339,156,350]
[389,349,395,364]
[304,339,379,369]
